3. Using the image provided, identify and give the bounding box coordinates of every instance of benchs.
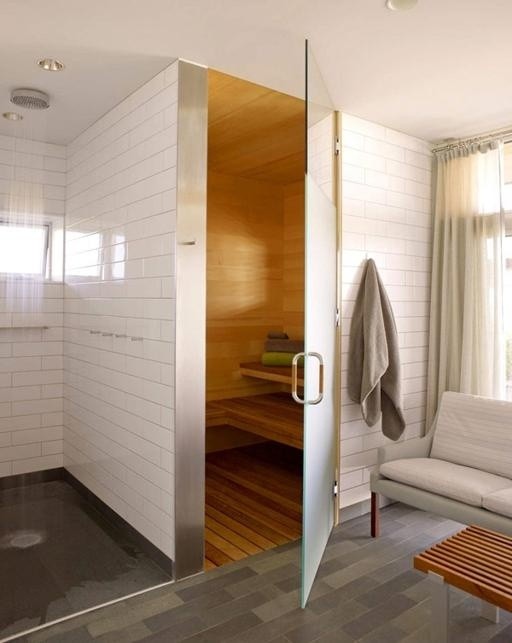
[206,392,304,447]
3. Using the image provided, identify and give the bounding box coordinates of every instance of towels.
[359,258,406,441]
[261,332,304,367]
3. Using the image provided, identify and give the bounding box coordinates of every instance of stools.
[413,524,512,643]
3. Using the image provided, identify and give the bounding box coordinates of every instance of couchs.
[369,391,512,538]
